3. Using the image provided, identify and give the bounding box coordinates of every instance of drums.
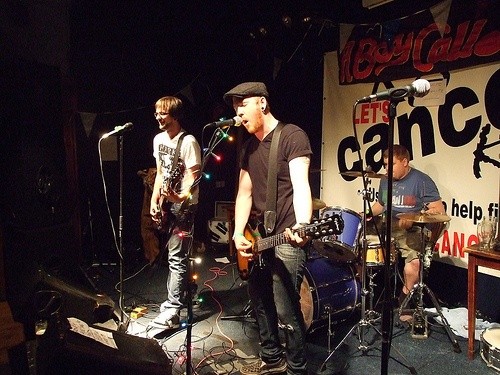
[312,205,363,264]
[361,240,396,267]
[299,249,362,334]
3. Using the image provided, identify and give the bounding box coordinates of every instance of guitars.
[152,160,186,232]
[236,212,345,282]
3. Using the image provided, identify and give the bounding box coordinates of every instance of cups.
[476,216,499,252]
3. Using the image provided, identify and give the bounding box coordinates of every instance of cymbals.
[338,171,387,179]
[312,198,327,211]
[396,212,452,224]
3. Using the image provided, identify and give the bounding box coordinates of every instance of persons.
[358,145,445,322]
[150,97,201,326]
[223,83,312,375]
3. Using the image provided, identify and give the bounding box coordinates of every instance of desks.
[462,243,500,361]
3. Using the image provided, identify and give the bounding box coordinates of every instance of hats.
[223,82,268,105]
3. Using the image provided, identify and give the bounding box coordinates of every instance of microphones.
[100,122,134,141]
[207,115,242,127]
[357,78,430,104]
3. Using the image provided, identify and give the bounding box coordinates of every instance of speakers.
[22,267,171,375]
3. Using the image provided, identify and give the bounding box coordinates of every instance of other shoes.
[397,298,413,322]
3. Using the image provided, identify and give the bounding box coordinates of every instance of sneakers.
[159,304,189,316]
[240,358,287,375]
[153,308,180,329]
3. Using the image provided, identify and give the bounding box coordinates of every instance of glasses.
[154,112,170,117]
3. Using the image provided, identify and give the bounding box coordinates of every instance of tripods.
[319,176,461,374]
